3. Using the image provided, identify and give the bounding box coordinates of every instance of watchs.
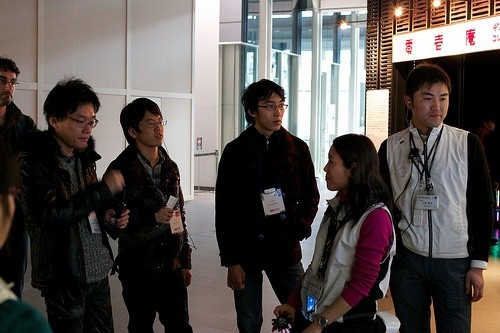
[313,314,330,329]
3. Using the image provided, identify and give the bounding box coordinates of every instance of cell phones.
[109,201,127,227]
[306,294,318,317]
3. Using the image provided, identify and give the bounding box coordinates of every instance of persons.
[375,64,496,333]
[273,133,397,333]
[214,78,321,333]
[0,57,130,333]
[102,95,194,333]
[476,115,500,246]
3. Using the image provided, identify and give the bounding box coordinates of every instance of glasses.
[140,120,167,128]
[66,116,98,128]
[0,79,20,87]
[257,104,288,111]
